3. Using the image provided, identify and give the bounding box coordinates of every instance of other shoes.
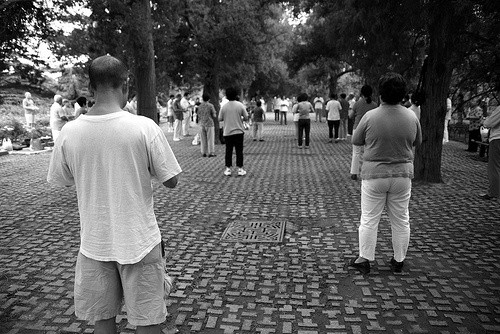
[478,194,497,200]
[173,137,182,141]
[296,143,310,149]
[328,137,347,143]
[259,139,264,142]
[253,138,257,141]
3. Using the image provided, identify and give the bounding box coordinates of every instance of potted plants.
[0,119,53,151]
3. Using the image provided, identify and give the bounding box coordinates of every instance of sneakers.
[224,168,246,175]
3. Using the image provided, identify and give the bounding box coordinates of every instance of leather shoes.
[202,153,216,158]
[390,257,404,273]
[351,174,357,180]
[350,257,370,274]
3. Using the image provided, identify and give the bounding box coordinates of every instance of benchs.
[471,128,491,162]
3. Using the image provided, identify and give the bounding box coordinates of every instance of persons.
[47,56,184,334]
[23,86,500,199]
[351,73,422,274]
